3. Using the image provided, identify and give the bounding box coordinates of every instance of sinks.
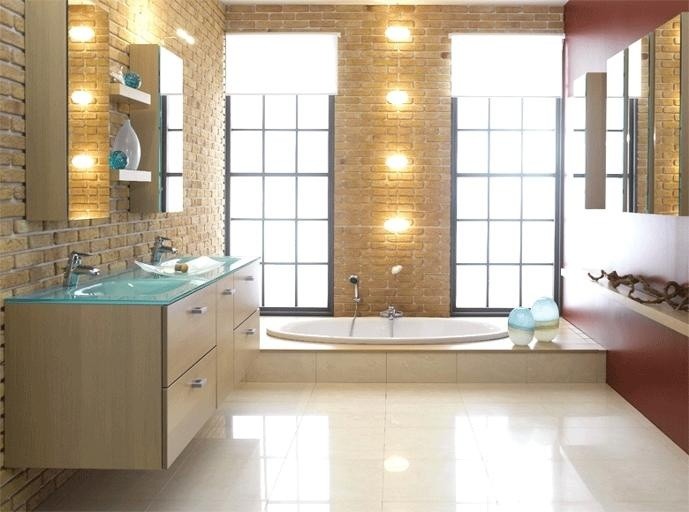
[181,255,242,267]
[69,278,189,296]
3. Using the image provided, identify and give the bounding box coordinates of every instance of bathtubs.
[266,315,511,344]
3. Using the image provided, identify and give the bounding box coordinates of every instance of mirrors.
[603,13,680,217]
[66,3,109,224]
[158,46,185,215]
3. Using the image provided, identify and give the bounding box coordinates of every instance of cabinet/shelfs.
[107,83,154,182]
[217,275,235,407]
[24,0,110,224]
[234,263,262,391]
[4,284,219,471]
[129,43,185,215]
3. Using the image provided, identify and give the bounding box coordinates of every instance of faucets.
[150,235,177,265]
[380,306,404,318]
[63,249,101,287]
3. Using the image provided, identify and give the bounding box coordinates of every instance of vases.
[507,296,561,347]
[114,119,141,170]
